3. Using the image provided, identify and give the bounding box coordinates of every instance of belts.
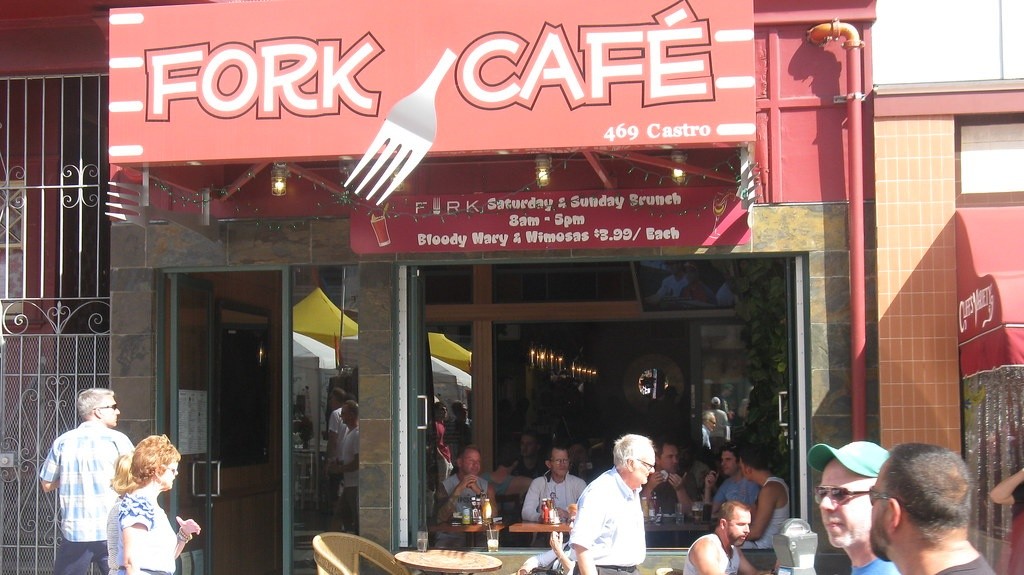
[595,565,636,573]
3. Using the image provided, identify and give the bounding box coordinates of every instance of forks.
[343,49,458,206]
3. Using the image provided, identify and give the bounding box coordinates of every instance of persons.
[700,410,716,448]
[684,501,781,575]
[112,434,202,575]
[573,434,657,575]
[711,397,729,443]
[479,429,792,549]
[426,400,470,480]
[988,467,1024,575]
[518,504,578,575]
[298,387,361,536]
[436,445,496,546]
[806,439,900,575]
[870,442,1000,575]
[39,389,136,575]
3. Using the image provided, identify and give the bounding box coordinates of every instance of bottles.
[471,497,492,524]
[642,496,658,521]
[541,498,555,524]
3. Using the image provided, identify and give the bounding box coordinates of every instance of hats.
[808,441,890,477]
[712,397,720,406]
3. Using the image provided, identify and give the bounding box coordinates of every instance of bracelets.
[177,527,193,543]
[476,490,484,498]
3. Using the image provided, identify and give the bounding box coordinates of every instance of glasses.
[93,404,117,411]
[165,467,176,474]
[870,486,916,517]
[552,459,571,465]
[814,486,870,503]
[636,459,656,469]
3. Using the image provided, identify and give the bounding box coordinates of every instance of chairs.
[313,531,410,575]
[655,567,683,575]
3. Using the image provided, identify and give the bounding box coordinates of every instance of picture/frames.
[495,324,521,341]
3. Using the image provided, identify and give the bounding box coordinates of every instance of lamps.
[270,161,289,197]
[527,341,597,384]
[256,340,266,367]
[670,149,686,184]
[392,162,405,191]
[535,155,551,189]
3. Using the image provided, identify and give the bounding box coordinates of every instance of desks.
[394,548,502,575]
[508,523,571,546]
[433,524,505,546]
[643,520,719,547]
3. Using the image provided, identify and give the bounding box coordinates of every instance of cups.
[675,503,683,524]
[692,501,703,524]
[487,529,498,552]
[417,531,428,552]
